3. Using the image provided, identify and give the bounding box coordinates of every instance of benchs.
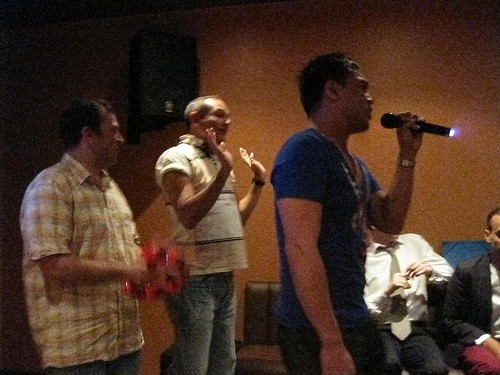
[236,282,470,375]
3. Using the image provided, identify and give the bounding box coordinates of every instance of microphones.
[380,113,451,137]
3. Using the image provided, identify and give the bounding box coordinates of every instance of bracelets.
[397,159,415,167]
[251,178,265,186]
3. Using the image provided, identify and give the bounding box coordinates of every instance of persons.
[362,216,456,375]
[441,208,500,375]
[18,97,167,375]
[270,51,426,375]
[153,96,267,375]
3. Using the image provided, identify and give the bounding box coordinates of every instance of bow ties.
[377,242,413,341]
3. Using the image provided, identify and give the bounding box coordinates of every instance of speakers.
[129,29,198,119]
